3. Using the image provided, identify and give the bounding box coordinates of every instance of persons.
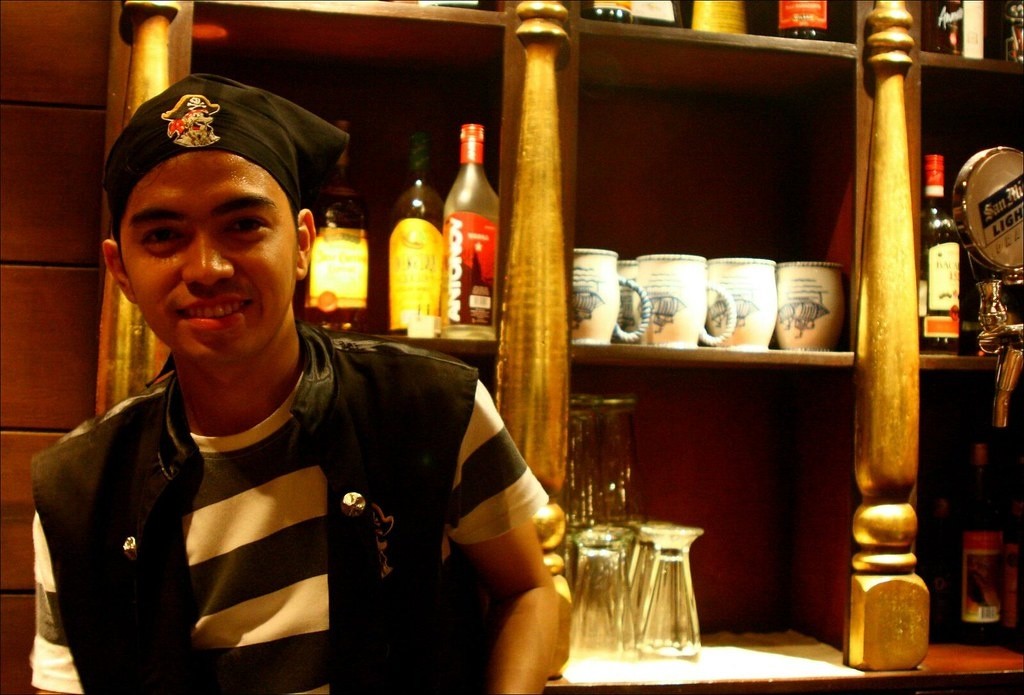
[30,73,560,695]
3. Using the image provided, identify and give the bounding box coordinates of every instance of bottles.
[441,121,501,338]
[583,0,844,38]
[923,435,1023,654]
[285,118,369,340]
[564,391,702,663]
[919,155,963,353]
[382,132,444,336]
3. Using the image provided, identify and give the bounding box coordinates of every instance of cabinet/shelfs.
[96,0,1023,695]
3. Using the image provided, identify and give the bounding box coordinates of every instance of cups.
[569,248,652,342]
[776,261,846,352]
[707,255,776,353]
[616,260,737,349]
